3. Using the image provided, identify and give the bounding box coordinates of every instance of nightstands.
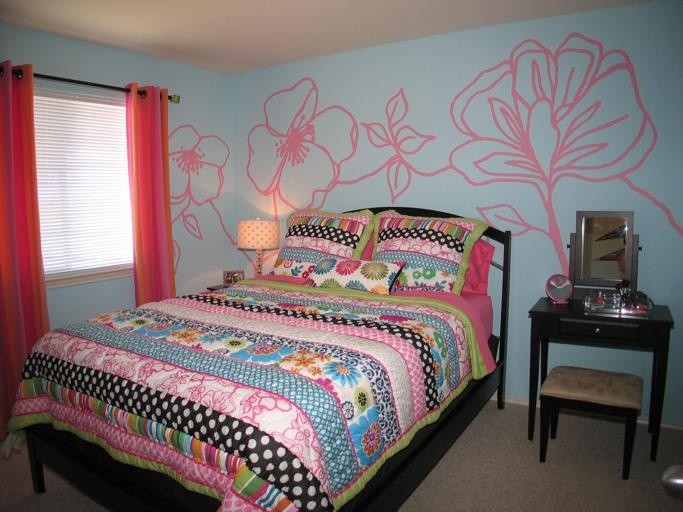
[207,284,224,291]
[528,297,675,448]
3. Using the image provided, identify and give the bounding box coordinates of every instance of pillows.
[274,210,376,284]
[371,210,488,296]
[305,256,405,296]
[463,239,495,298]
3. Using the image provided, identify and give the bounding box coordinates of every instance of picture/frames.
[223,269,245,288]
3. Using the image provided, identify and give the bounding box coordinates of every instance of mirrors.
[567,211,643,301]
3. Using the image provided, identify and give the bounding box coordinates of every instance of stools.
[539,365,644,449]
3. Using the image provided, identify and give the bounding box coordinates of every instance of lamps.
[236,219,280,278]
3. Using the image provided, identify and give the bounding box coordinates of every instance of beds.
[20,207,512,510]
